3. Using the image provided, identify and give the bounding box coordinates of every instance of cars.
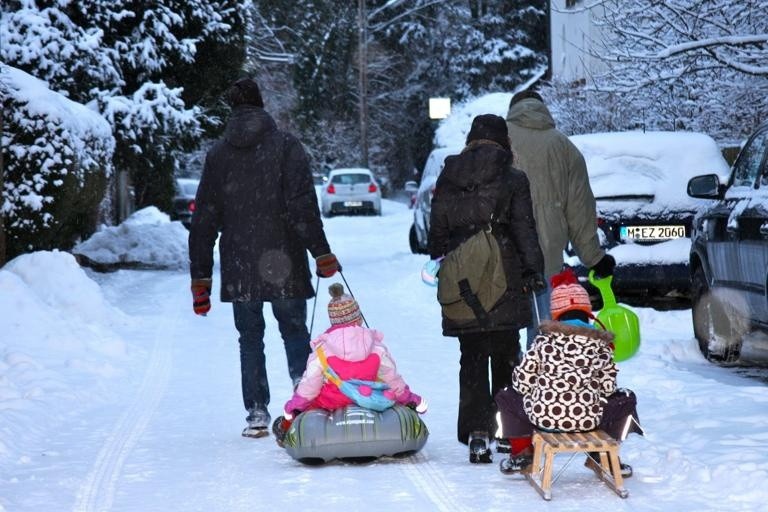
[716,138,762,186]
[352,163,391,199]
[100,176,201,229]
[320,168,383,217]
[688,120,767,369]
[406,147,465,254]
[562,131,753,312]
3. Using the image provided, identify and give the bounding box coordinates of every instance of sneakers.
[468,431,535,478]
[273,416,292,447]
[242,402,272,437]
[585,455,630,476]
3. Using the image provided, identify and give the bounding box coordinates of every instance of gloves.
[396,385,429,414]
[191,277,212,317]
[285,391,312,419]
[315,253,342,277]
[590,254,616,278]
[519,269,548,297]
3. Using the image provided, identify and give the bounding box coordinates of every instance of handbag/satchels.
[339,379,396,412]
[437,230,510,327]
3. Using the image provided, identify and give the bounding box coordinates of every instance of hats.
[328,283,361,326]
[549,268,606,332]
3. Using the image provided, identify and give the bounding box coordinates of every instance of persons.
[492,270,645,476]
[429,113,548,463]
[271,283,422,445]
[505,92,617,361]
[187,78,341,438]
